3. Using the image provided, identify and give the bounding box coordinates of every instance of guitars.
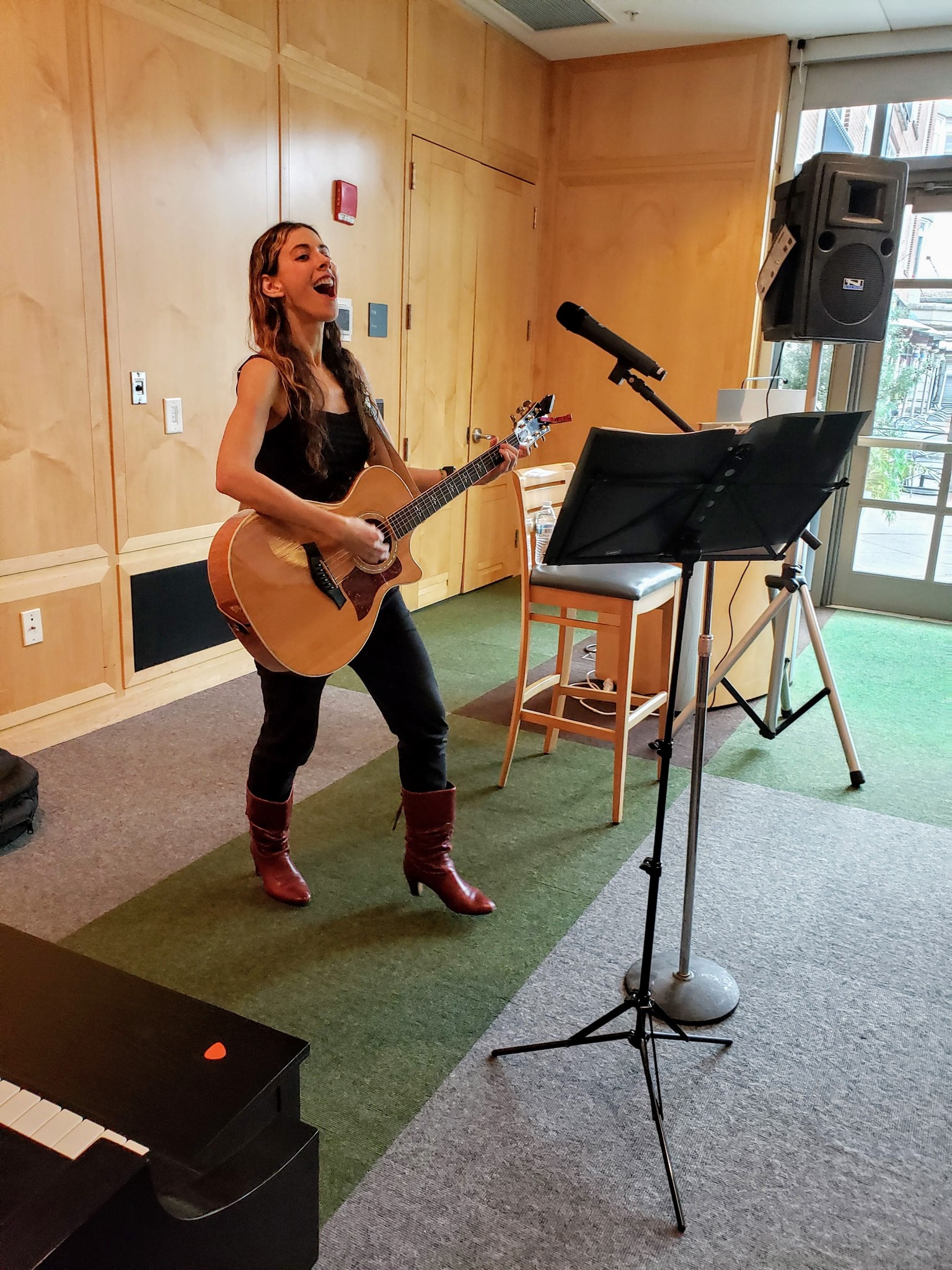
[206,392,572,678]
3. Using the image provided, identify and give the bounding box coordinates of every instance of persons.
[215,221,531,916]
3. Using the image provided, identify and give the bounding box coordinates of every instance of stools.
[495,461,682,827]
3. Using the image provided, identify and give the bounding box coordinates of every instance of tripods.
[668,337,860,785]
[492,409,872,1229]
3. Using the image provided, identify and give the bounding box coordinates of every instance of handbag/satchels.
[0,748,39,849]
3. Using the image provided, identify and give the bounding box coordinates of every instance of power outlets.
[130,371,147,405]
[163,397,183,435]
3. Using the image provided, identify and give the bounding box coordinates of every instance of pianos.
[0,921,322,1270]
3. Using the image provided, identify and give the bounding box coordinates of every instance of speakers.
[764,151,909,345]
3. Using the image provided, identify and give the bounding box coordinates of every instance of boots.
[243,773,312,906]
[392,781,496,916]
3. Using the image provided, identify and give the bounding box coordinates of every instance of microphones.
[554,301,667,382]
